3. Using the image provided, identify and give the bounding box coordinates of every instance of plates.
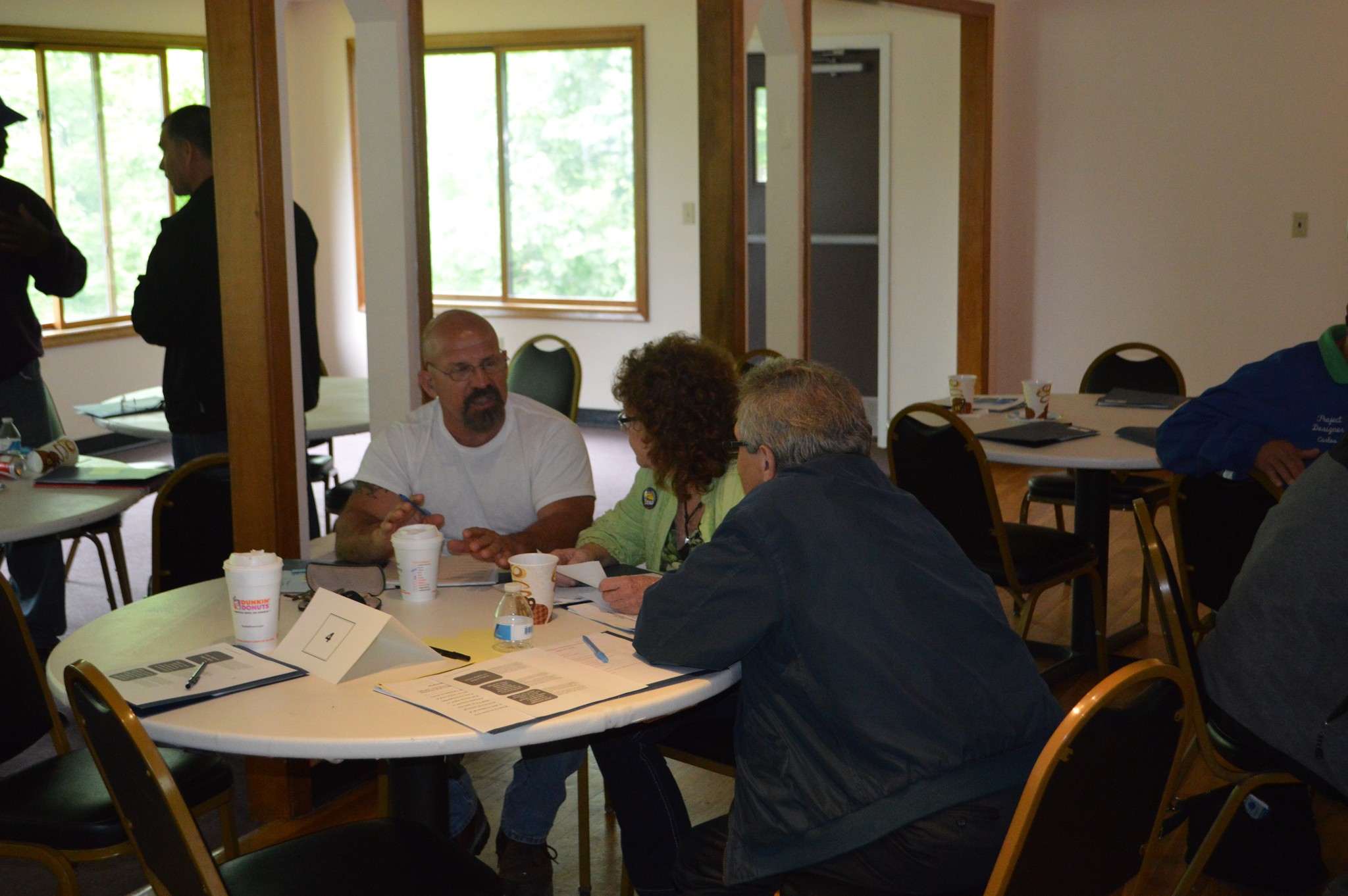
[1005,410,1064,421]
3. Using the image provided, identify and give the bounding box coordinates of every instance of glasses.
[617,411,642,431]
[721,437,750,453]
[425,348,509,383]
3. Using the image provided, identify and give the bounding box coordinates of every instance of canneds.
[0,454,28,480]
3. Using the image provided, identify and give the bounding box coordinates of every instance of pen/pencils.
[186,659,208,689]
[399,493,431,517]
[429,646,471,662]
[582,635,609,663]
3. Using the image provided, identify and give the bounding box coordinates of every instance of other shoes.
[494,823,557,896]
[459,798,492,855]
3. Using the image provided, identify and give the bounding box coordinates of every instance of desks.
[46,554,742,841]
[91,375,370,539]
[0,455,150,611]
[909,394,1191,685]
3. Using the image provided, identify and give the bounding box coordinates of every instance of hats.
[0,98,26,127]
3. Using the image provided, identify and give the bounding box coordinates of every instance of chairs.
[888,344,1304,896]
[0,331,582,896]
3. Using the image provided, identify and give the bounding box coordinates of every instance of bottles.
[0,417,22,455]
[493,581,534,653]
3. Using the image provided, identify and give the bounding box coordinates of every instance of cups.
[390,523,445,602]
[507,552,559,625]
[947,375,978,415]
[1021,380,1053,419]
[26,434,80,473]
[223,548,284,652]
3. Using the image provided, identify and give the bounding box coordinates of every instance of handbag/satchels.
[1159,779,1322,896]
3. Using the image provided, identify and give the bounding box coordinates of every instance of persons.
[131,100,321,601]
[0,97,84,743]
[321,311,594,895]
[1151,310,1348,807]
[541,333,1062,895]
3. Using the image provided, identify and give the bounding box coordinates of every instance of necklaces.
[678,499,703,544]
